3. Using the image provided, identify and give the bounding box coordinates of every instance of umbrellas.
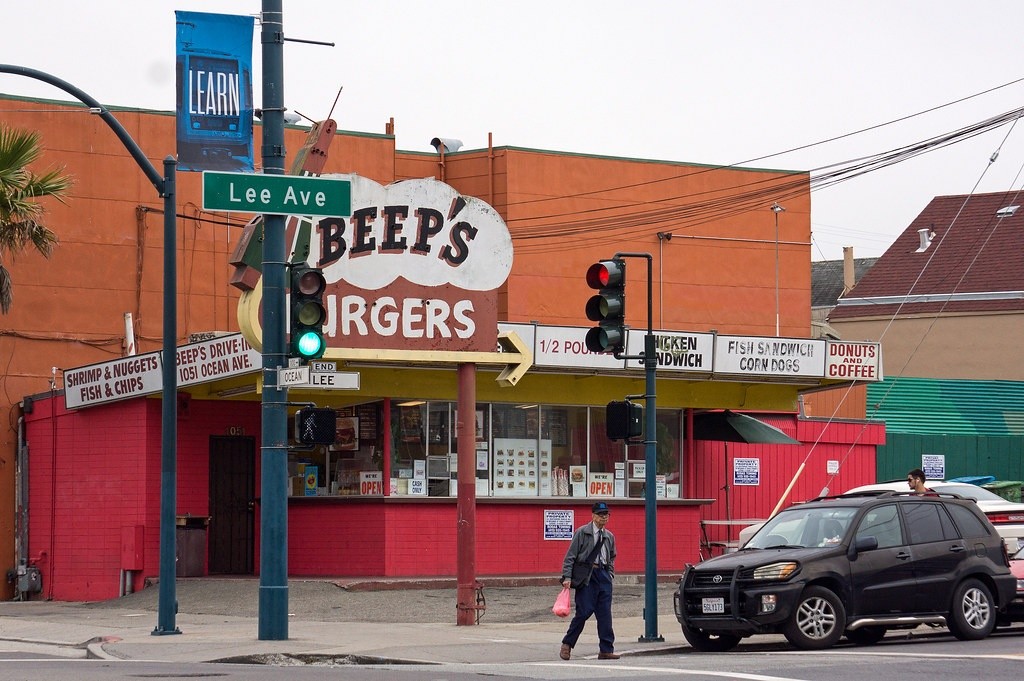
[667,410,802,518]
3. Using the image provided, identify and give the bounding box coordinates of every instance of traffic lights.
[585,258,626,353]
[288,263,329,363]
[605,399,644,441]
[294,404,337,447]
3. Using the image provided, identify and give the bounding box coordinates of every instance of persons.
[907,469,939,497]
[558,502,620,660]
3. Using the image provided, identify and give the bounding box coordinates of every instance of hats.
[591,502,612,515]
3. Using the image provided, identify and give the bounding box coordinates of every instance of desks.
[701,518,768,555]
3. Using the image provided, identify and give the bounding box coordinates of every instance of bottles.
[297,459,312,477]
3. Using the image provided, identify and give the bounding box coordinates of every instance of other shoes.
[559,643,572,660]
[598,652,620,660]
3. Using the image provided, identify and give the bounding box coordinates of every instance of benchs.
[701,542,740,554]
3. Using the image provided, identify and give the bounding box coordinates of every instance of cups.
[528,460,534,467]
[528,451,534,457]
[563,470,569,496]
[558,470,564,495]
[334,428,356,450]
[518,468,525,477]
[541,450,547,458]
[552,468,558,495]
[497,469,504,476]
[507,481,514,488]
[529,480,535,489]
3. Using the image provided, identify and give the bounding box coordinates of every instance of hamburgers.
[334,419,357,450]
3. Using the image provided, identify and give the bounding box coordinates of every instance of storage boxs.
[289,476,305,497]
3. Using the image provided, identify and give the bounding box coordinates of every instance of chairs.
[819,515,915,541]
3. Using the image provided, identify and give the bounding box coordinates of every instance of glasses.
[908,477,915,482]
[593,512,611,517]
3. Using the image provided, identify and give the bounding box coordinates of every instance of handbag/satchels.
[551,586,571,618]
[559,561,593,591]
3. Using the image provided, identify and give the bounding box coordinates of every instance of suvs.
[673,488,1018,652]
[737,478,1024,626]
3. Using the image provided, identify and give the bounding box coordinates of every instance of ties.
[597,529,603,560]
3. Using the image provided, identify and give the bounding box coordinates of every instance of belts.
[592,563,604,569]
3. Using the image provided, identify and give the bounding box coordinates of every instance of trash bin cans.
[947,477,995,487]
[980,480,1024,503]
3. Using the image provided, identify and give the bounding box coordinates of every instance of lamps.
[657,231,673,241]
